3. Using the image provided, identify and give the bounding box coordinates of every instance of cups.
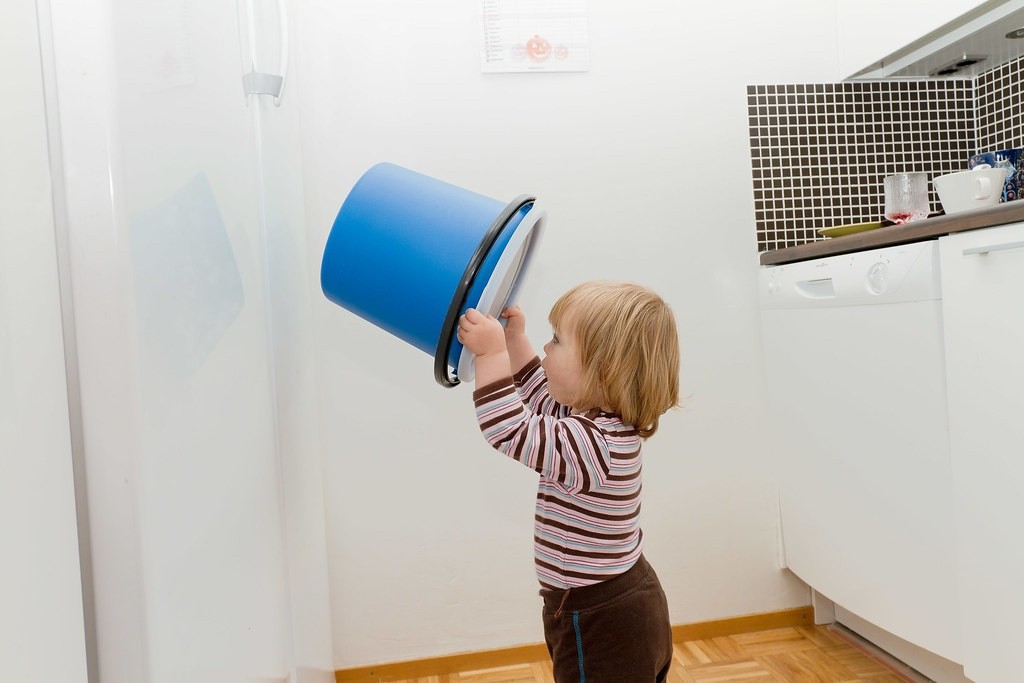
[883,173,931,224]
[931,167,1008,214]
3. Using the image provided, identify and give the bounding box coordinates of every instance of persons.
[457,280,679,683]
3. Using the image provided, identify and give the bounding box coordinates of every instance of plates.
[817,221,884,239]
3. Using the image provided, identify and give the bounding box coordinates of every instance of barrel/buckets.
[320,161,547,392]
